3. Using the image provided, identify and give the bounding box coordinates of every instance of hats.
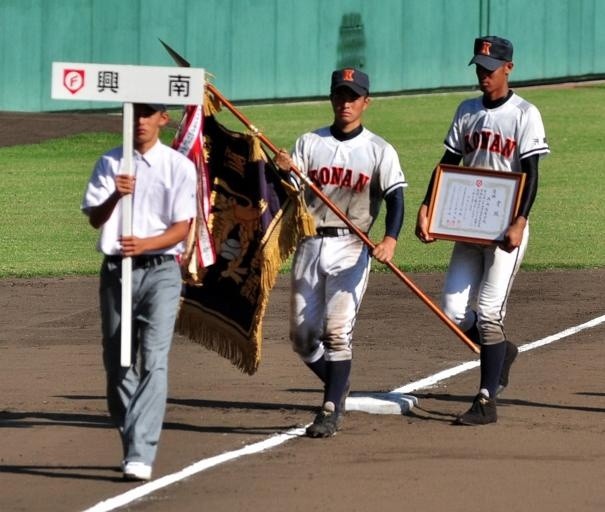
[469,35,513,72]
[131,104,165,112]
[330,67,369,96]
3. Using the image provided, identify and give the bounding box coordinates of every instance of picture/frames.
[426,164,527,246]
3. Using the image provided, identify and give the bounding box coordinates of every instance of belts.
[111,253,173,268]
[317,227,351,238]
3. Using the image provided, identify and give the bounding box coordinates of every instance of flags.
[171,95,316,377]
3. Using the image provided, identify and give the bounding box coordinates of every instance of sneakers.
[493,339,517,392]
[452,394,498,424]
[124,462,152,480]
[308,414,337,437]
[333,379,351,426]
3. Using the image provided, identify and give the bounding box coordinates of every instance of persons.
[271,67,410,438]
[413,34,550,427]
[79,102,198,485]
